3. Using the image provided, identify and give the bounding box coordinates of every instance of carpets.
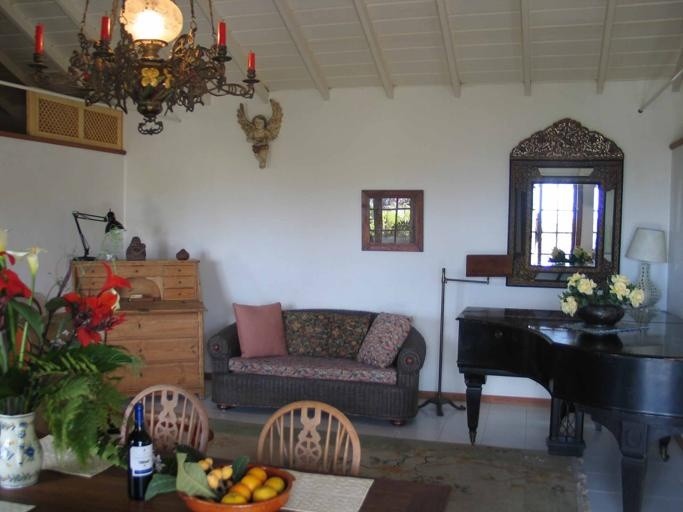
[129,416,591,511]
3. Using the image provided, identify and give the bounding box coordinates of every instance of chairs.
[120,385,209,455]
[256,401,360,477]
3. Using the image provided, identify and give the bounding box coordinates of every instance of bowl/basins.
[178,464,296,512]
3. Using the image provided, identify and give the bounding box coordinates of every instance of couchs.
[207,309,426,425]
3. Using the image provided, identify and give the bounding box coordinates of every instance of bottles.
[127,403,154,499]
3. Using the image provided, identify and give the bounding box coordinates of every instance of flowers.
[0,229,140,460]
[549,247,592,265]
[560,273,644,317]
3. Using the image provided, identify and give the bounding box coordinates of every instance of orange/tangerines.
[222,466,284,504]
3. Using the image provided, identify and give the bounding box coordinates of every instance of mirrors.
[506,120,624,290]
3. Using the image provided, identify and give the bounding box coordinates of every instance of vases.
[580,305,624,326]
[0,413,41,489]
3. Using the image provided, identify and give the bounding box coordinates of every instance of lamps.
[27,1,259,135]
[625,227,668,307]
[72,209,127,262]
[121,0,182,46]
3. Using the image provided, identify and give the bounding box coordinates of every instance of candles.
[217,19,227,46]
[35,24,44,51]
[248,51,255,70]
[100,15,109,42]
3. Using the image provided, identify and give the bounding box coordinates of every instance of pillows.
[356,311,414,368]
[232,302,288,357]
